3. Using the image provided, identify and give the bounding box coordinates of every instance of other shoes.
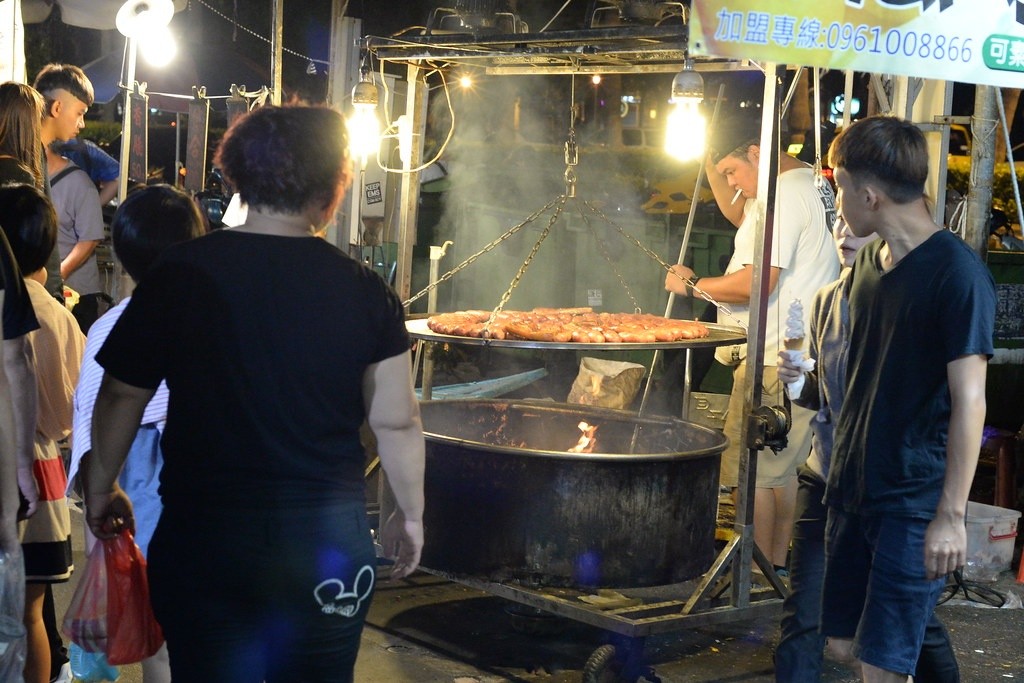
[777,569,788,577]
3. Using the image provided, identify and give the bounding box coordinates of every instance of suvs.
[821,119,1024,178]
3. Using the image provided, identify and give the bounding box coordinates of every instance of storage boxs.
[965,501,1022,572]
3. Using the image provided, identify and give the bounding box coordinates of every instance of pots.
[415,398,729,589]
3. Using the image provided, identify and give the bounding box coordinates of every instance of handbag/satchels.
[61,527,164,665]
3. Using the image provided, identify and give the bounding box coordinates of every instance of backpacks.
[70,292,115,337]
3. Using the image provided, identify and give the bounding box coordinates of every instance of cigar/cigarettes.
[731,189,743,205]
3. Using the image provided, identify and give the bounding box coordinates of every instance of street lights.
[592,74,603,140]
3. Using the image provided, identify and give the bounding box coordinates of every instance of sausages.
[428,306,710,342]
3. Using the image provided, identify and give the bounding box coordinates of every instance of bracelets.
[685,275,702,297]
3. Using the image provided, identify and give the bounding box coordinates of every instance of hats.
[710,116,757,165]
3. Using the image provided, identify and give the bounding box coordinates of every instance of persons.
[0,63,206,683]
[86,106,425,683]
[664,110,842,579]
[772,113,994,683]
